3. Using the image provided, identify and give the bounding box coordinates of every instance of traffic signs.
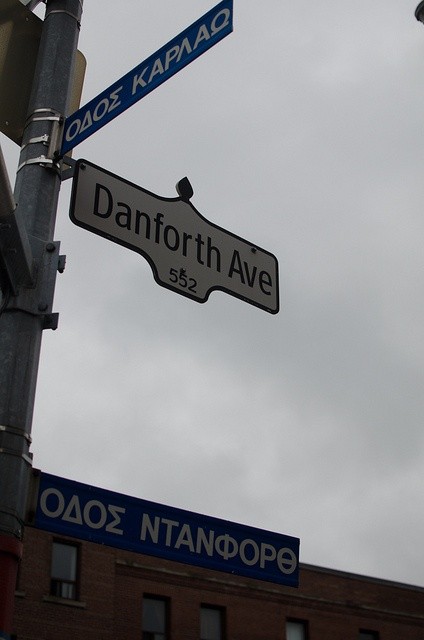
[69,158,279,314]
[34,473,299,589]
[61,0,233,155]
